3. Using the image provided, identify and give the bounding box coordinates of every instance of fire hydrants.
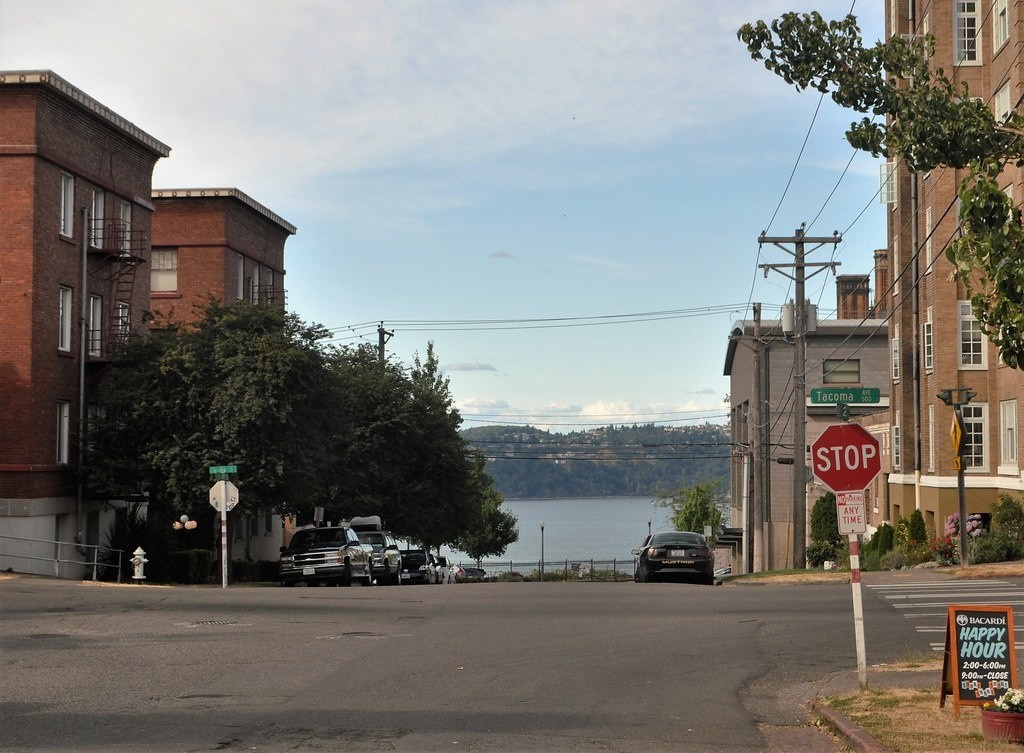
[129,545,149,583]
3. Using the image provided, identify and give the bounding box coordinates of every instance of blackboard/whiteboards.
[941,605,1018,705]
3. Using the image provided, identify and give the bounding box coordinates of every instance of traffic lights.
[966,392,977,404]
[936,392,948,403]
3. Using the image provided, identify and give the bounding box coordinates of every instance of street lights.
[647,517,651,536]
[540,521,545,582]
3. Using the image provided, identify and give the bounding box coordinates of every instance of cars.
[631,532,714,585]
[429,554,455,584]
[455,567,489,583]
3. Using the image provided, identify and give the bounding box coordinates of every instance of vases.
[982,710,1024,742]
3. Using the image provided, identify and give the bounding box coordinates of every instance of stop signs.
[810,423,882,492]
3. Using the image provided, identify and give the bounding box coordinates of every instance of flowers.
[982,688,1024,713]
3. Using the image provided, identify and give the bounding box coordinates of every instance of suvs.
[350,516,403,585]
[399,550,431,585]
[280,526,374,586]
